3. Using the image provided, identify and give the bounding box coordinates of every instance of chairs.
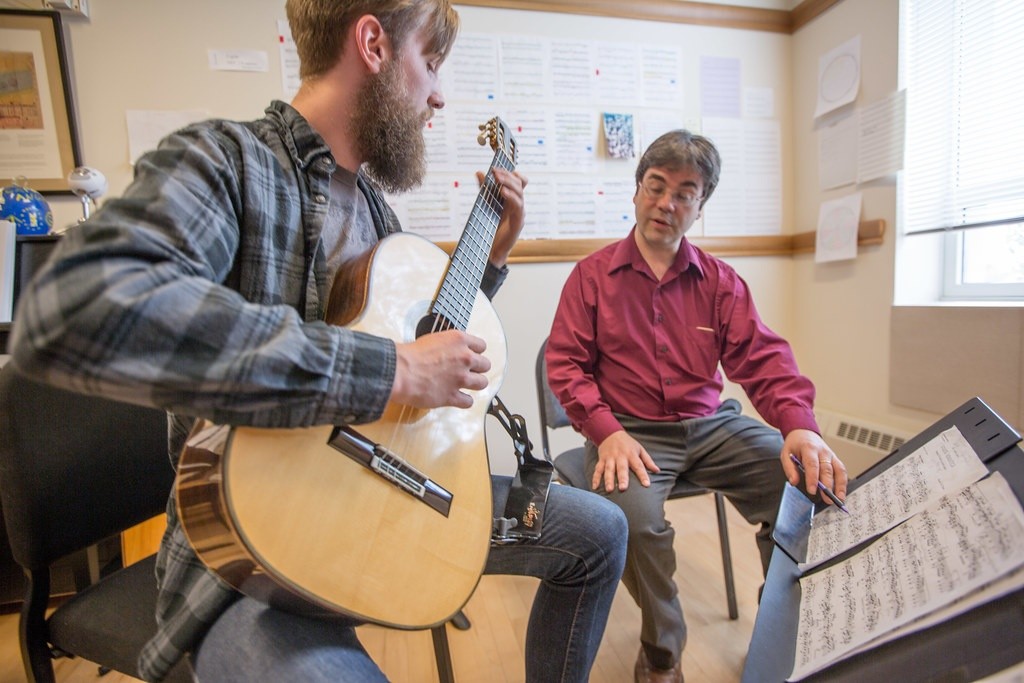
[533,337,739,622]
[0,360,456,682]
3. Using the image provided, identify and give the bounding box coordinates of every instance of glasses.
[637,180,704,206]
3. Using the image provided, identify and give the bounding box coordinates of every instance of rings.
[819,460,831,464]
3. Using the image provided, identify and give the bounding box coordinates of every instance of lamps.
[67,165,108,223]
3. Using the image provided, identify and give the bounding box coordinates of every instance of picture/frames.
[0,8,84,196]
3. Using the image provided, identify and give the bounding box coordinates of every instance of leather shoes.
[633,642,685,683]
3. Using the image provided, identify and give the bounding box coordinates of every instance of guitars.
[172,113,521,631]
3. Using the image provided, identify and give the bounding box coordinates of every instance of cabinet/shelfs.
[0,235,124,614]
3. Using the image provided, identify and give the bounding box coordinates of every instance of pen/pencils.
[789,451,851,516]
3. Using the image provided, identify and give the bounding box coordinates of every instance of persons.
[547,129,847,682]
[6,0,629,683]
